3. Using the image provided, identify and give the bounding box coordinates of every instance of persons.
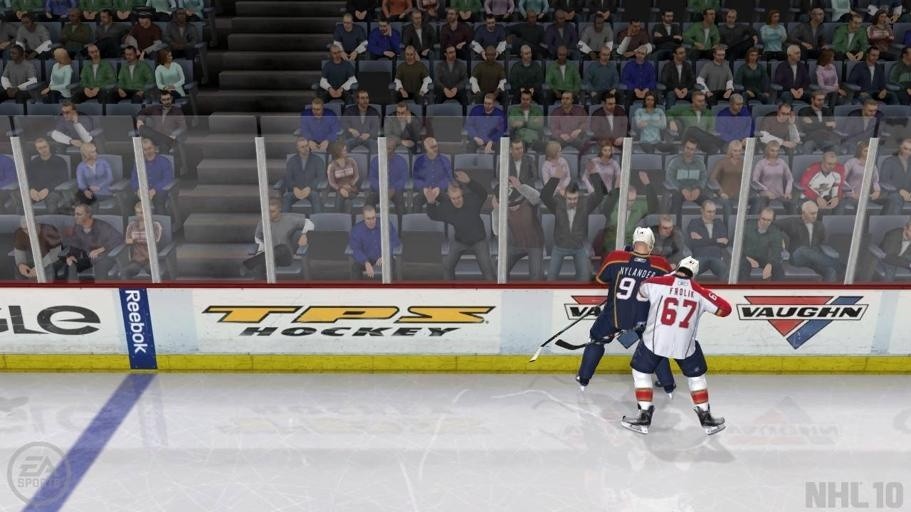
[577,224,677,394]
[1,0,202,283]
[623,254,733,426]
[245,1,911,287]
